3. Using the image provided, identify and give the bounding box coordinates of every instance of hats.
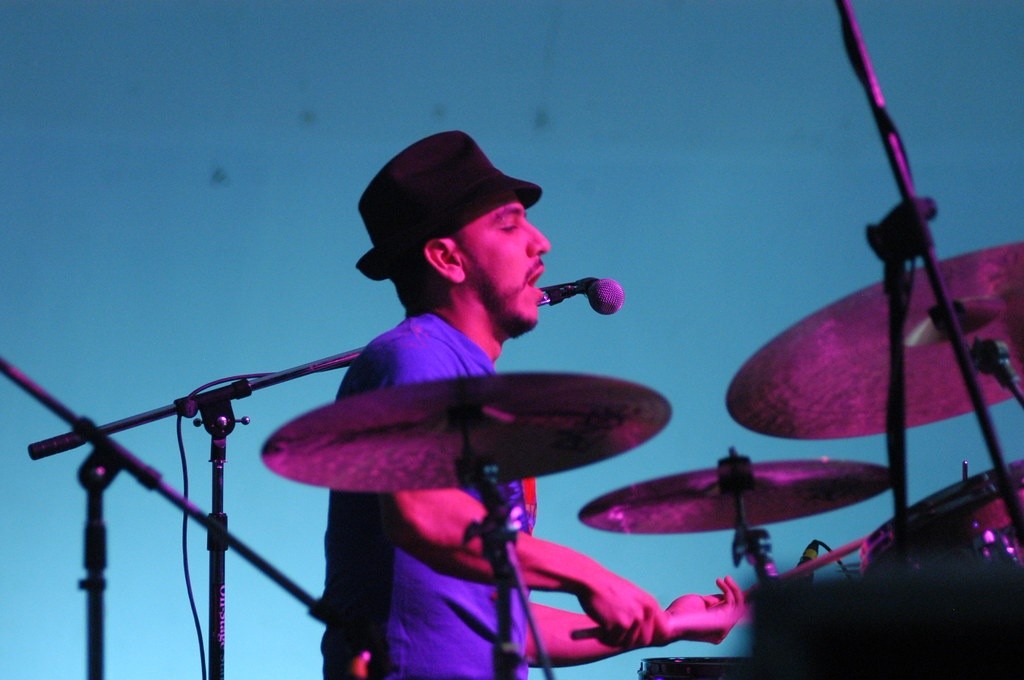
[355,130,543,281]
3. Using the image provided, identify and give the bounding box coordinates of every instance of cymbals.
[725,240,1024,442]
[260,373,672,494]
[578,461,892,534]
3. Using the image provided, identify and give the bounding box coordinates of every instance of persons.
[320,131,747,680]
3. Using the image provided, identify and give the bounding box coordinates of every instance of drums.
[860,457,1023,575]
[639,654,751,680]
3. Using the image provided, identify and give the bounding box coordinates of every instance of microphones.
[578,276,624,314]
[793,539,820,587]
